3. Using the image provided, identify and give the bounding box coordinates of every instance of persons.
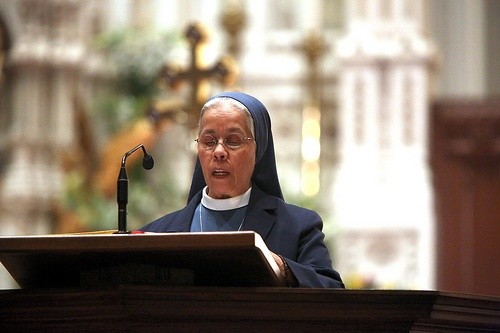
[134,92,344,288]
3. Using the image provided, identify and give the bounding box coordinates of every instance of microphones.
[113,144,154,235]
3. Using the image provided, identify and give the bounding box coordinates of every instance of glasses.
[195,133,256,149]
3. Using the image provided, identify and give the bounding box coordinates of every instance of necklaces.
[199,203,244,231]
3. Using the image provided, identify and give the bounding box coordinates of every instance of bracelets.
[278,254,289,283]
